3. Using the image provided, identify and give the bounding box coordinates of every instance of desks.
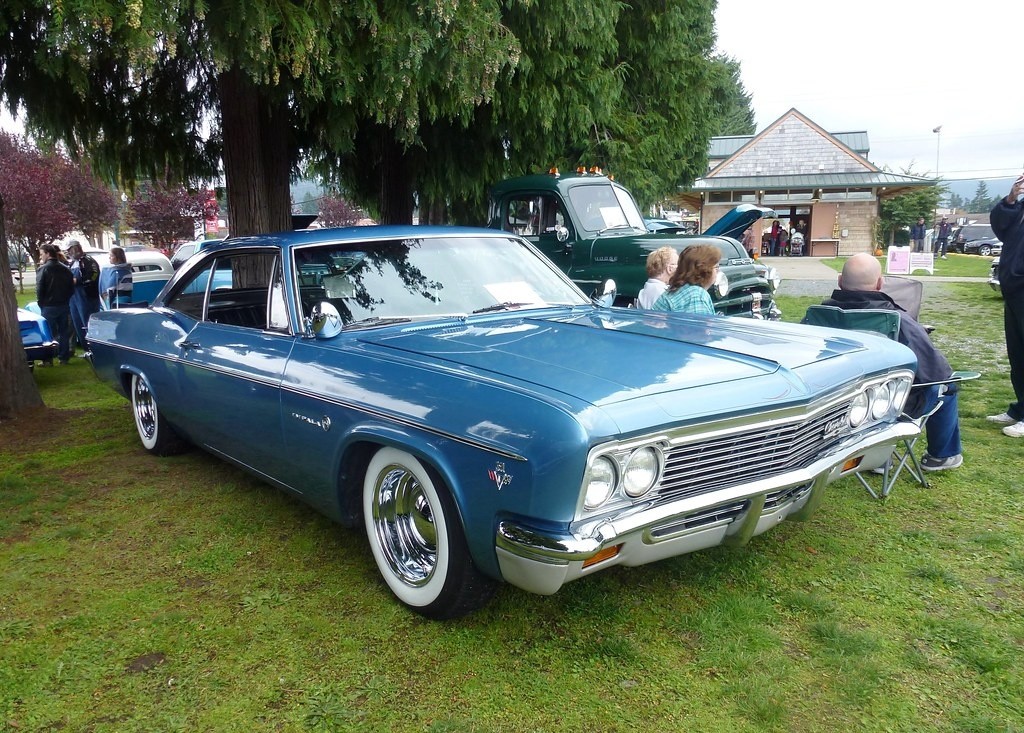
[811,239,840,258]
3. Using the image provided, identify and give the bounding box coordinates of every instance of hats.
[942,217,949,221]
[65,240,79,250]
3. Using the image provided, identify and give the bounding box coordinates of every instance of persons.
[910,218,926,252]
[36,240,100,366]
[795,219,808,256]
[652,245,721,315]
[986,173,1024,438]
[770,220,788,256]
[636,246,679,309]
[933,218,952,259]
[101,248,132,308]
[821,252,963,471]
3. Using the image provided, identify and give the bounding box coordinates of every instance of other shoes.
[60,359,68,365]
[779,254,781,257]
[78,353,91,357]
[783,254,787,256]
[38,359,53,367]
[934,256,938,259]
[940,255,947,259]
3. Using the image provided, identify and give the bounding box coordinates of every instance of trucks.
[486,165,783,324]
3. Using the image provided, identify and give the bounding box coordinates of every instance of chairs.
[881,276,936,336]
[807,304,981,508]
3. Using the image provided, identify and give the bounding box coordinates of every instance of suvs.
[929,223,1000,291]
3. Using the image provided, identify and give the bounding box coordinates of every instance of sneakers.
[1002,420,1024,437]
[870,455,892,473]
[986,411,1016,422]
[920,448,964,472]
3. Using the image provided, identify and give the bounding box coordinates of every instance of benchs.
[209,296,349,330]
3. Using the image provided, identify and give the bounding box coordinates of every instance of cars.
[16,236,366,373]
[638,202,779,241]
[82,224,922,621]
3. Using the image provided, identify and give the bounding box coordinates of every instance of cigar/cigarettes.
[1015,178,1024,183]
